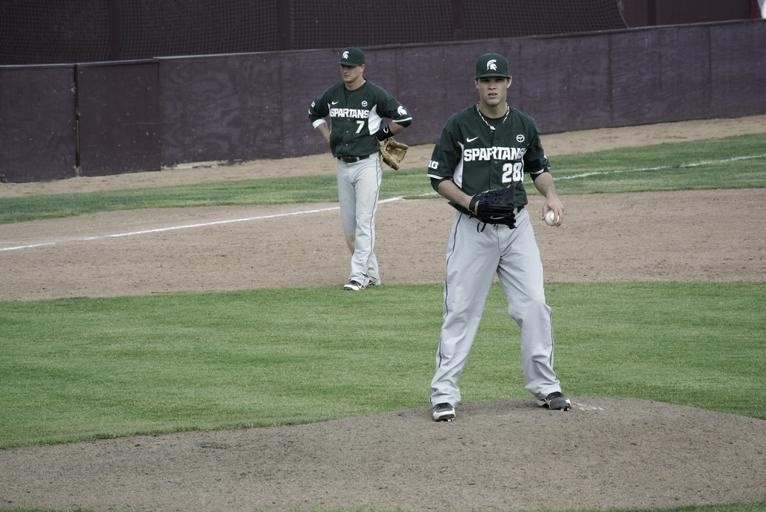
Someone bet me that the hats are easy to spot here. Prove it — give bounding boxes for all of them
[338,48,365,66]
[475,54,510,79]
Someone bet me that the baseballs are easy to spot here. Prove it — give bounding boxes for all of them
[545,210,559,226]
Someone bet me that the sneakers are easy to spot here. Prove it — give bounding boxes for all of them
[344,280,380,291]
[432,402,456,422]
[537,392,570,409]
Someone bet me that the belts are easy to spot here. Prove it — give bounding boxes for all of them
[517,206,524,213]
[337,155,369,163]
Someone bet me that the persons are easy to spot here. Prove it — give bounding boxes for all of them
[427,52,571,422]
[307,47,413,292]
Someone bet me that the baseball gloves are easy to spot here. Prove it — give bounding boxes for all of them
[468,188,516,224]
[377,137,408,170]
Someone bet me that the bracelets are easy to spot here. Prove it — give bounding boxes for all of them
[376,124,394,141]
